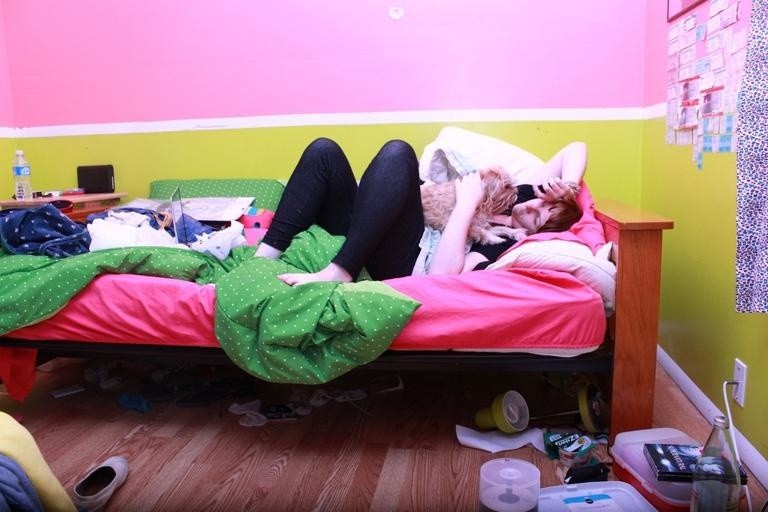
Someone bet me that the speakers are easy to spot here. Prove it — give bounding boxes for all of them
[77,164,115,194]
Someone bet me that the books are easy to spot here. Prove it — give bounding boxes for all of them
[641,443,750,487]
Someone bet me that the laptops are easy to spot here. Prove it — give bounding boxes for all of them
[170,186,188,245]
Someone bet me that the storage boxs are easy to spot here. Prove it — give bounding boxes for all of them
[538,427,747,511]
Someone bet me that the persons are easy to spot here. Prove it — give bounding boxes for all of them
[254,138,588,290]
[0,410,76,511]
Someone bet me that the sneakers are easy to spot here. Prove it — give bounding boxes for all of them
[72,455,128,512]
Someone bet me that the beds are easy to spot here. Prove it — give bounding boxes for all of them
[0,172,678,448]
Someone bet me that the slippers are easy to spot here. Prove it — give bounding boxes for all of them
[176,381,239,408]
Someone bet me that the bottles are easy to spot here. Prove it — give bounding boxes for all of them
[688,415,742,512]
[12,150,34,200]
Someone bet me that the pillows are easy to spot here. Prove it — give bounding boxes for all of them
[484,238,616,318]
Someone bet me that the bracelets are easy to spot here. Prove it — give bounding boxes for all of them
[567,183,582,195]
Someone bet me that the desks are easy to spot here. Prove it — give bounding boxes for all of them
[2,191,130,223]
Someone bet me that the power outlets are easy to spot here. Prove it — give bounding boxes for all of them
[730,357,748,409]
